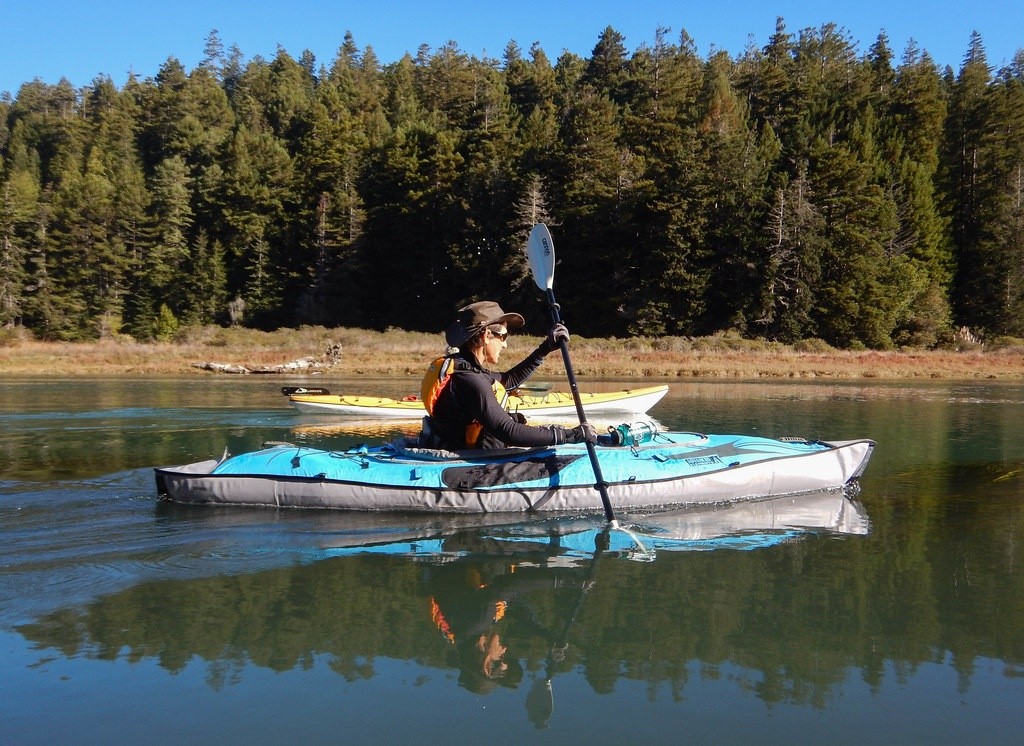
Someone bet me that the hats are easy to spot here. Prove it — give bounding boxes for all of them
[445,300,526,348]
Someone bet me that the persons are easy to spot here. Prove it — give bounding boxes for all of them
[416,300,597,453]
[428,552,599,697]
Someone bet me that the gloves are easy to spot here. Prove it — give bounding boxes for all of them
[539,320,570,358]
[553,421,598,446]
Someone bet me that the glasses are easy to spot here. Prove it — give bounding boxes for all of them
[491,329,510,342]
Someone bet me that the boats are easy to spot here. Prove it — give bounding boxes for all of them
[280,384,672,419]
[155,427,875,513]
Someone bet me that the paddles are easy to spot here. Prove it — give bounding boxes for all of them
[526,222,652,553]
[515,380,553,394]
